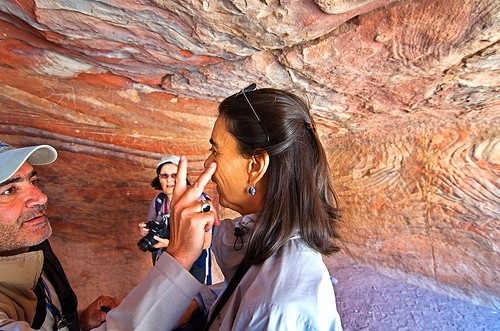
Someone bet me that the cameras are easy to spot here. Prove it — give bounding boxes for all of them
[137,220,170,252]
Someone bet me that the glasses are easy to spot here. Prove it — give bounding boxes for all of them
[158,173,176,179]
[235,83,270,144]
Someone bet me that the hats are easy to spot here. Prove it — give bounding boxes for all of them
[157,155,180,167]
[0,140,58,185]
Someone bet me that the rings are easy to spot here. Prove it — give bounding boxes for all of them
[201,200,210,212]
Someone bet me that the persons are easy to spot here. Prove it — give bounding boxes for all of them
[139,157,212,286]
[0,140,220,331]
[177,87,343,331]
